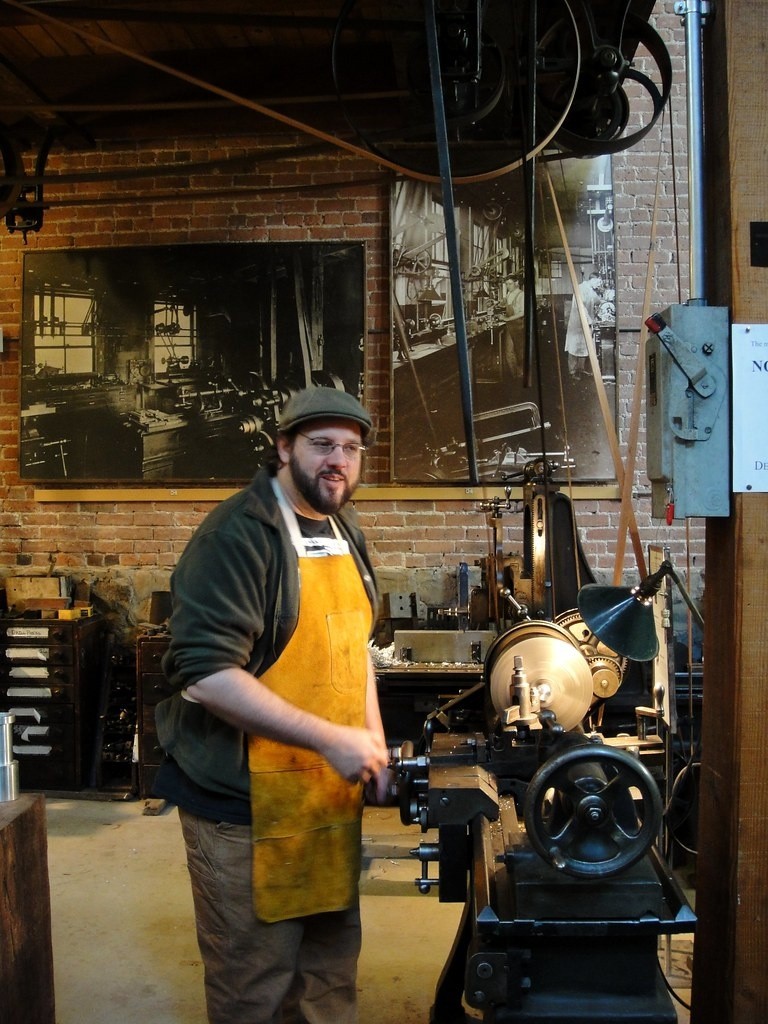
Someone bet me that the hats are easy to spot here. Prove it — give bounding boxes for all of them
[277,387,373,436]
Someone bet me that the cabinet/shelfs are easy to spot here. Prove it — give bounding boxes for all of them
[137,626,173,798]
[0,613,111,791]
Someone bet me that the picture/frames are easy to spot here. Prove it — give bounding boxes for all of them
[18,240,364,484]
[390,150,618,483]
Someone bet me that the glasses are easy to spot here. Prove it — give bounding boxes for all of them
[297,430,370,459]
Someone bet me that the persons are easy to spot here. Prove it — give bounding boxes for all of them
[146,384,392,1024]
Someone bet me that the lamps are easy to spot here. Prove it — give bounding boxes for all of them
[577,560,704,661]
